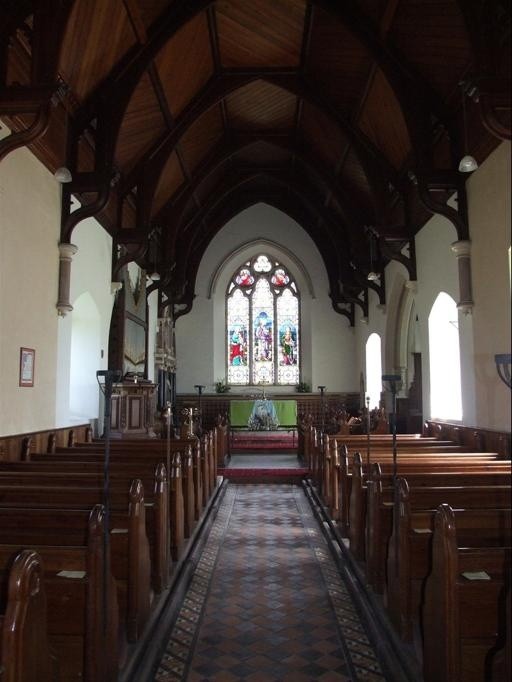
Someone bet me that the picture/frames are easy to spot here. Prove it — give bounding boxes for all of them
[19,345,36,387]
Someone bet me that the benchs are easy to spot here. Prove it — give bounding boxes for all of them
[0,428,218,682]
[304,426,512,682]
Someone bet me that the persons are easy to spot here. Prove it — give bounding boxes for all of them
[280,326,294,367]
[255,317,271,361]
[229,326,245,366]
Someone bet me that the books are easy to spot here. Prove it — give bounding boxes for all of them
[461,568,492,581]
[413,527,433,534]
[110,527,129,534]
[145,501,155,507]
[383,501,395,506]
[57,569,86,579]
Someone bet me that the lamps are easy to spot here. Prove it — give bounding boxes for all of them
[458,85,479,173]
[367,231,378,281]
[55,103,72,183]
[151,236,161,281]
